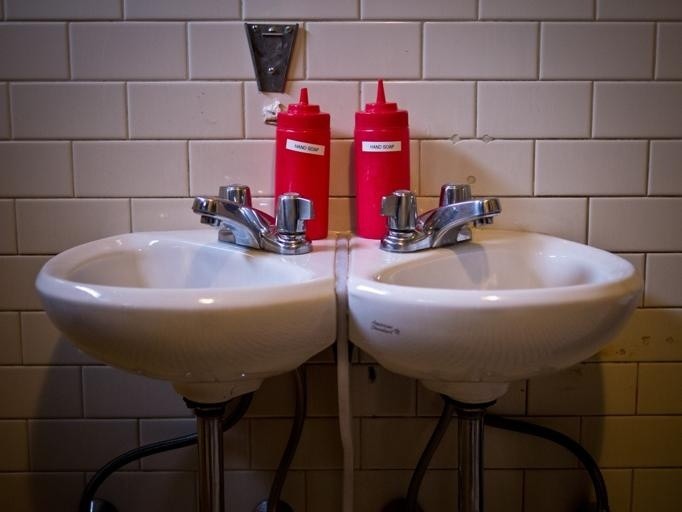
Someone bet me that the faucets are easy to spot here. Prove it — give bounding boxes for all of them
[190,182,314,252]
[377,182,500,252]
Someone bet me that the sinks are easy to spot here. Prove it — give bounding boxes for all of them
[32,226,335,382]
[346,234,632,355]
